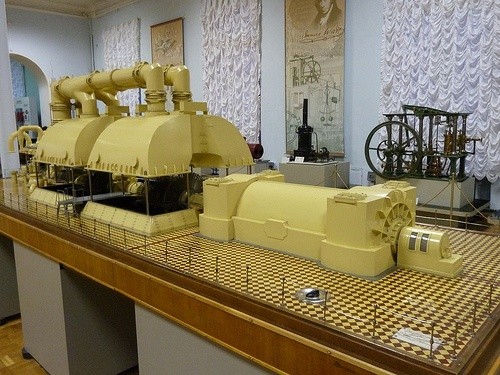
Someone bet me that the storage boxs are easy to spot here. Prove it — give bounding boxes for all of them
[376,173,476,209]
[219,162,268,177]
[279,162,349,189]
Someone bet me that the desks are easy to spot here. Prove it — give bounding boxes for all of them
[414,199,491,227]
[1,177,500,375]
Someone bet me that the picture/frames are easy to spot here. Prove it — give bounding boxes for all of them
[150,17,185,67]
